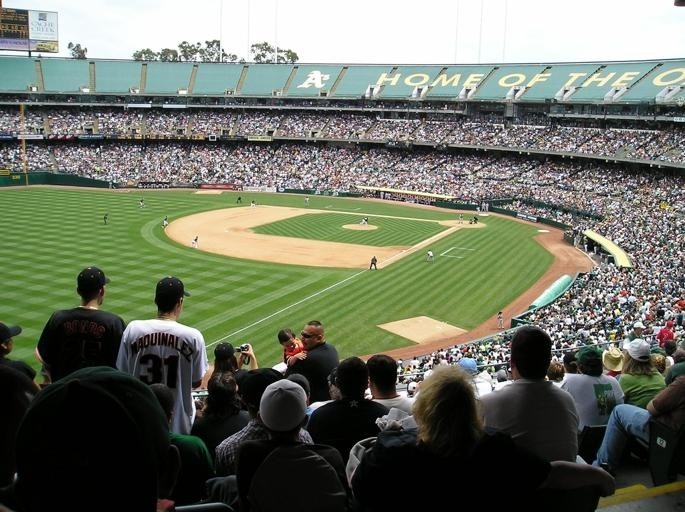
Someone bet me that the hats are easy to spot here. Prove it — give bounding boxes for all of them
[1,267,235,361]
[563,321,674,377]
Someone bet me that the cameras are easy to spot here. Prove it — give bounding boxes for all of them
[236,344,249,352]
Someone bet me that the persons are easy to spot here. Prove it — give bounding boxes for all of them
[0,94,685,512]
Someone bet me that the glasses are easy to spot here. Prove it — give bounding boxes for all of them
[300,330,319,338]
